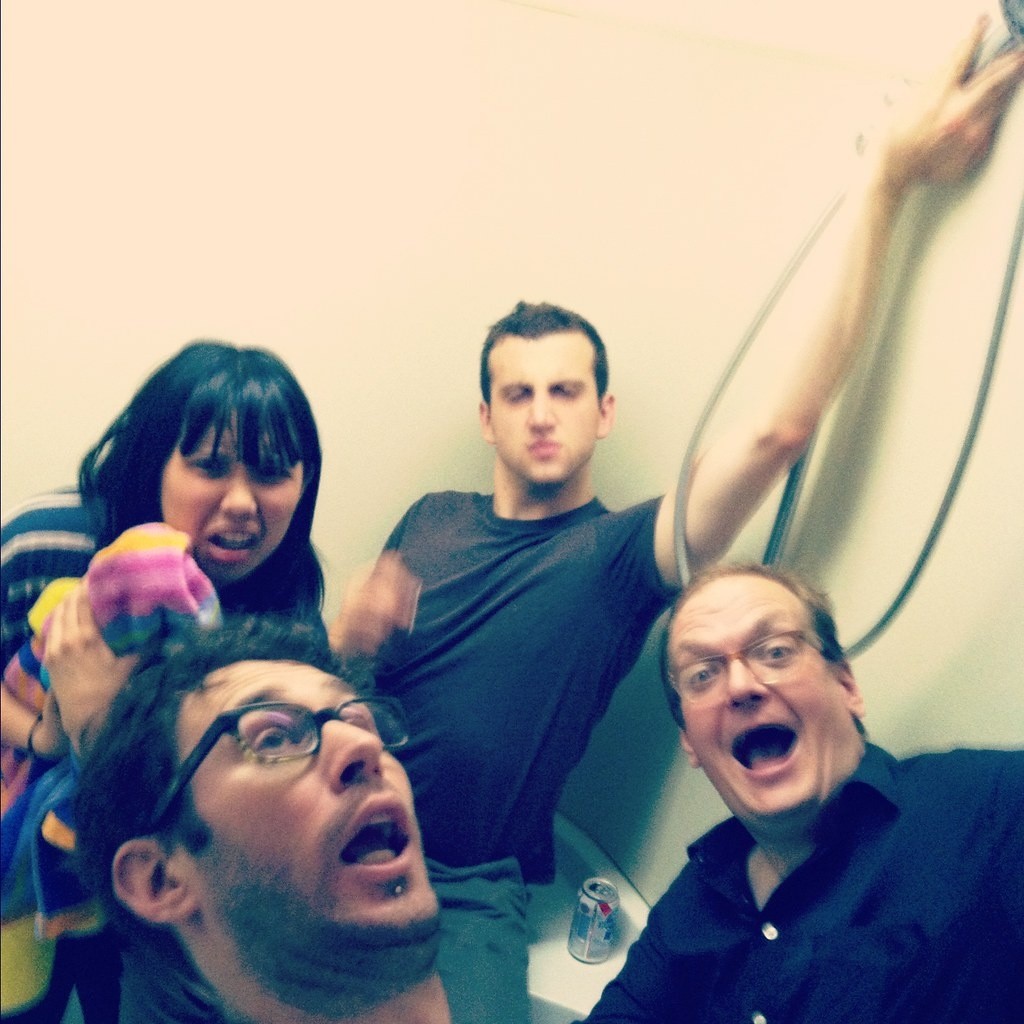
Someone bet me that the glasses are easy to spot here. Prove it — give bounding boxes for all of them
[145,697,413,832]
[671,632,834,700]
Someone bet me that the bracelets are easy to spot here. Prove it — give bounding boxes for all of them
[27,713,44,754]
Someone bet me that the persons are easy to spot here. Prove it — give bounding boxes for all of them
[329,16,1024,1020]
[571,561,1024,1023]
[71,612,457,1023]
[0,337,332,927]
